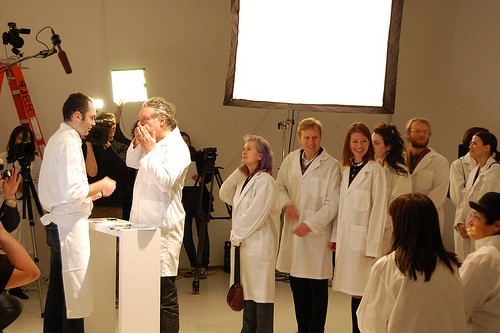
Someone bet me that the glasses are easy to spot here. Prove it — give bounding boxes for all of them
[408,128,430,135]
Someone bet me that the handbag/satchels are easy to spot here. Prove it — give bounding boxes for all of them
[227,282,244,311]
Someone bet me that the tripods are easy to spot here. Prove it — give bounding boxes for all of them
[190,169,232,294]
[18,168,48,317]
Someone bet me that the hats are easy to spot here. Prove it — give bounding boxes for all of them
[469,192,499,222]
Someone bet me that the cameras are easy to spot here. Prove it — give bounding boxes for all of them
[0,159,12,180]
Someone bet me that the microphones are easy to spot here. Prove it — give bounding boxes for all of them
[50,29,72,74]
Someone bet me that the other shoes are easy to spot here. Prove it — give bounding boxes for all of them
[199,268,208,278]
[9,287,28,299]
[184,266,199,277]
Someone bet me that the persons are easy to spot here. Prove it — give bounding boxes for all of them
[455,129,500,264]
[38,94,120,333]
[217,131,282,333]
[271,116,343,333]
[331,121,393,333]
[402,118,450,245]
[368,125,414,208]
[123,97,193,333]
[128,113,213,279]
[450,125,500,264]
[355,193,469,333]
[0,127,47,333]
[451,193,500,333]
[81,108,137,309]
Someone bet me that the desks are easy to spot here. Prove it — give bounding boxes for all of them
[84,217,162,333]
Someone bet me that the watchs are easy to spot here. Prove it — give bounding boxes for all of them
[6,199,16,203]
